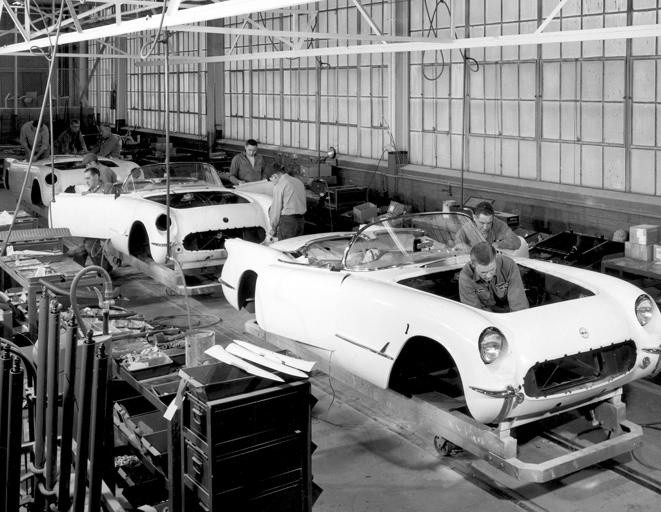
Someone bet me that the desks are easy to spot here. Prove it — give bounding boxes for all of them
[600,257,661,297]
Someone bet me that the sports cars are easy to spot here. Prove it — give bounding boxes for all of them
[2,153,144,219]
[216,212,660,431]
[48,161,271,279]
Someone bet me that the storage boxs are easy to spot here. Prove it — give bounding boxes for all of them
[621,223,661,265]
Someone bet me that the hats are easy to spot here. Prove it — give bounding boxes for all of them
[79,153,96,166]
[264,163,280,182]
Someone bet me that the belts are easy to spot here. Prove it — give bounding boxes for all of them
[281,214,303,218]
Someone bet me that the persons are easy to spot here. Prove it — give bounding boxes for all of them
[228,137,268,187]
[458,241,530,312]
[81,153,120,185]
[456,200,520,254]
[20,119,51,162]
[91,123,120,158]
[56,118,89,156]
[265,161,308,241]
[74,167,117,279]
